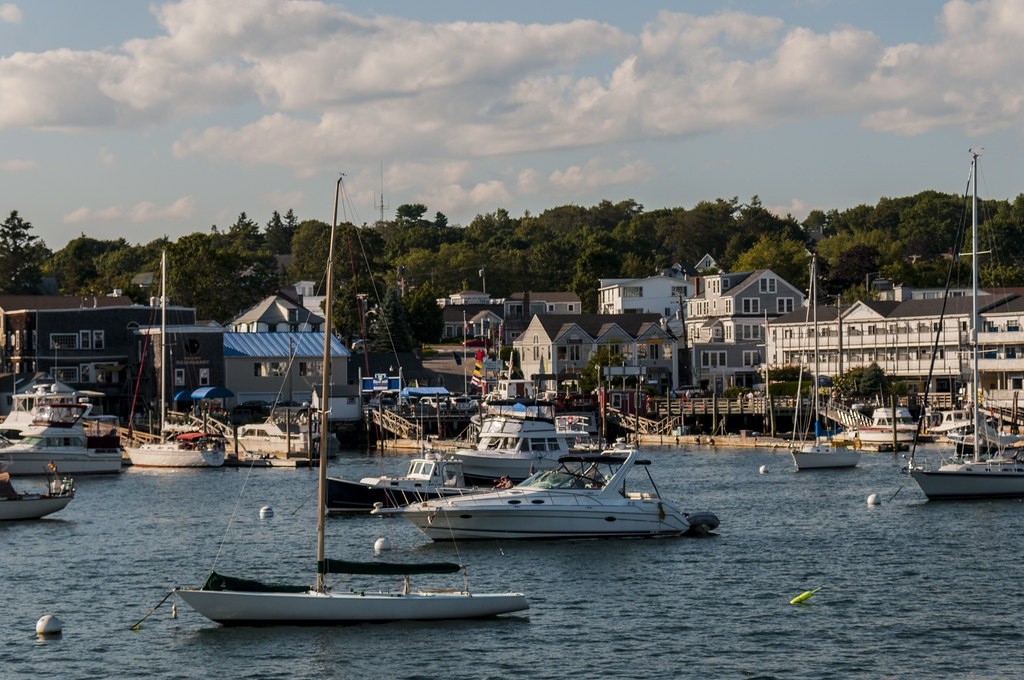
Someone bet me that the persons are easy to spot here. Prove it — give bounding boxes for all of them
[446,397,451,415]
[402,402,410,416]
[671,389,676,398]
[494,475,513,489]
[744,388,764,398]
[686,389,691,398]
[614,400,620,407]
[646,395,650,410]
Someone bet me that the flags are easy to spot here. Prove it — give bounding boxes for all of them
[475,351,484,363]
[464,317,469,335]
[499,325,503,337]
[471,375,482,385]
[453,351,461,365]
[473,362,482,375]
[530,463,534,476]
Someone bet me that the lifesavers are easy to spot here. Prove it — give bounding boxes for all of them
[39,408,44,413]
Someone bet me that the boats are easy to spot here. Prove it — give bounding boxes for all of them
[1,460,78,523]
[0,342,722,541]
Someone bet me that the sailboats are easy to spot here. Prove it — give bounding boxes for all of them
[784,250,866,470]
[833,150,1024,500]
[165,172,534,623]
